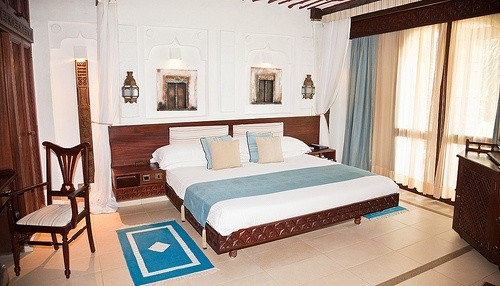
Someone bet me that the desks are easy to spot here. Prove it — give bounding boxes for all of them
[0,169,23,276]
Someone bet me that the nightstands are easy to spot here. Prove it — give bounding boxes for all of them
[311,147,336,160]
[112,164,165,201]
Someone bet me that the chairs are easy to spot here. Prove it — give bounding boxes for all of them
[15,141,95,280]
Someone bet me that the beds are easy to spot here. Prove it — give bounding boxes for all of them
[108,116,399,257]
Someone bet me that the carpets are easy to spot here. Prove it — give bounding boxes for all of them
[364,205,407,222]
[115,220,220,286]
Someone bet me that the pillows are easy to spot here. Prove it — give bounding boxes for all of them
[152,140,207,171]
[238,136,312,164]
[256,136,283,163]
[209,139,240,169]
[246,132,273,162]
[201,134,232,169]
[233,122,284,137]
[168,124,229,144]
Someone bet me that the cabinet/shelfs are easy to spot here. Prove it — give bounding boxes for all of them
[0,31,46,257]
[452,147,500,270]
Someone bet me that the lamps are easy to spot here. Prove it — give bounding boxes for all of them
[170,47,182,61]
[262,50,274,64]
[301,74,316,99]
[122,70,140,104]
